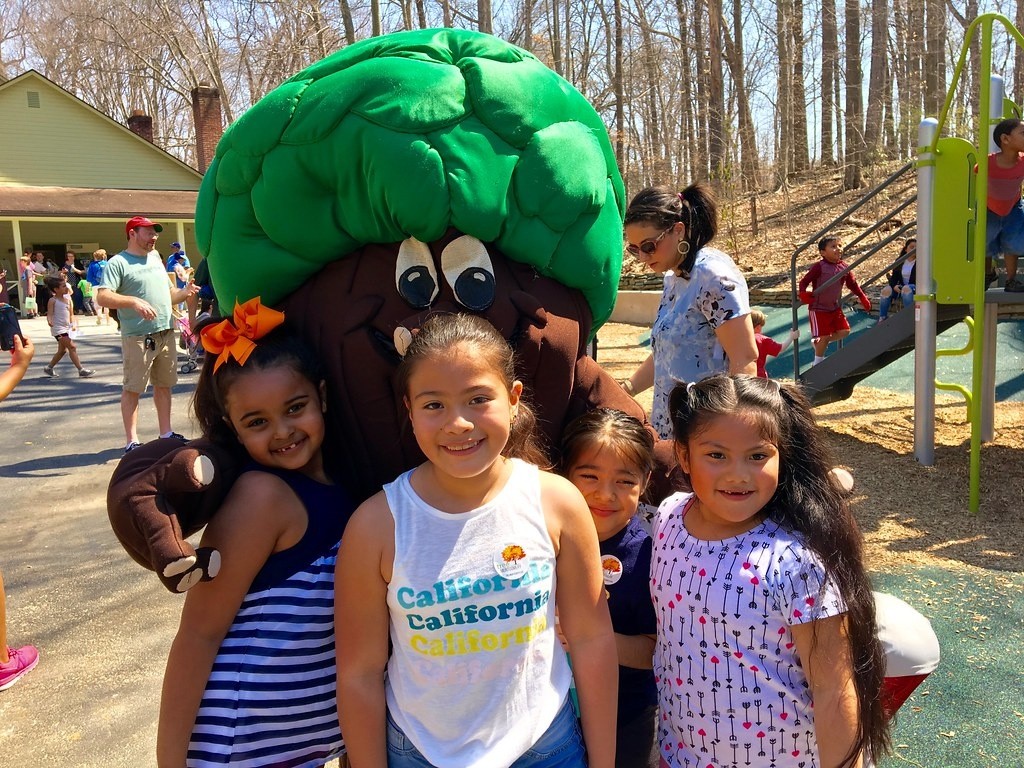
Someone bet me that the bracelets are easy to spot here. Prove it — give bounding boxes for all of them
[624,380,635,397]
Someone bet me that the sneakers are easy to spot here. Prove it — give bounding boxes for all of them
[1004,279,1024,292]
[44,367,59,377]
[0,645,39,694]
[79,368,95,378]
[985,267,998,290]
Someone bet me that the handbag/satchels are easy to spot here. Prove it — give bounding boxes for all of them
[25,296,35,309]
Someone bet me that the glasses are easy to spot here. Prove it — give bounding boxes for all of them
[136,228,159,238]
[626,223,675,256]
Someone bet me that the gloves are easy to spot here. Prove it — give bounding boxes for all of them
[799,292,816,304]
[859,294,871,312]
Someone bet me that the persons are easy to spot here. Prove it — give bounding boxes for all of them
[879,239,916,321]
[553,408,657,768]
[97,217,201,455]
[870,587,941,721]
[973,118,1024,292]
[800,233,872,367]
[155,296,346,767]
[750,310,800,379]
[0,302,39,691]
[43,277,95,378]
[334,314,619,767]
[0,242,110,325]
[623,182,759,440]
[160,242,213,355]
[649,372,893,768]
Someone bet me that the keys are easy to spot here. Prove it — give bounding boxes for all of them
[146,338,155,351]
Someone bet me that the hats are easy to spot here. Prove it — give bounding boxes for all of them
[174,252,186,259]
[170,242,180,249]
[125,217,163,234]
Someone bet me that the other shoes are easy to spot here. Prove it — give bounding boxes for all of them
[96,317,102,325]
[85,312,94,316]
[123,440,143,453]
[27,313,34,319]
[158,432,192,444]
[34,313,39,318]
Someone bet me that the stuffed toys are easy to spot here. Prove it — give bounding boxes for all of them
[106,26,687,594]
